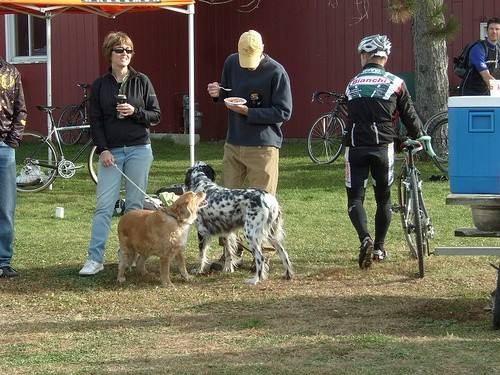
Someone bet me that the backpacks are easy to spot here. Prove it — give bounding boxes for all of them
[454,40,488,78]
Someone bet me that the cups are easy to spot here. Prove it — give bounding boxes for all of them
[56,206,64,219]
[489,80,500,97]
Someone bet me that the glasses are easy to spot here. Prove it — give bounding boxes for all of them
[112,47,132,54]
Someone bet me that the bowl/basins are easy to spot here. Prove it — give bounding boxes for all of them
[224,97,248,106]
[471,204,500,231]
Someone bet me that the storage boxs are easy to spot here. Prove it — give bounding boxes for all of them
[448,96,500,193]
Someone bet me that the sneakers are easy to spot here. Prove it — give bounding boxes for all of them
[372,248,387,261]
[250,257,270,272]
[0,266,18,279]
[211,254,243,273]
[79,260,104,275]
[118,258,136,270]
[359,236,373,269]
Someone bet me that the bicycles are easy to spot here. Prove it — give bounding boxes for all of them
[57,83,90,145]
[394,134,448,277]
[307,91,348,164]
[16,105,99,192]
[422,107,448,174]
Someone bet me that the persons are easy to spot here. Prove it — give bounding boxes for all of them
[343,34,427,271]
[206,30,293,274]
[79,31,162,275]
[0,56,27,276]
[459,18,500,98]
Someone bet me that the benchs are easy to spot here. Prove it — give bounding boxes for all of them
[454,227,499,237]
[434,247,500,256]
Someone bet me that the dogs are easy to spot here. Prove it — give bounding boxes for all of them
[117,191,209,287]
[185,162,296,285]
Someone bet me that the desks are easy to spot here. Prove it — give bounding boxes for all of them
[446,195,500,330]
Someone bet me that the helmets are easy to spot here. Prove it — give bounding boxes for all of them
[357,34,392,55]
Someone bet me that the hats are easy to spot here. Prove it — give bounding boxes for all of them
[238,30,263,69]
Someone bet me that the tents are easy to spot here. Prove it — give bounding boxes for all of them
[0,0,196,168]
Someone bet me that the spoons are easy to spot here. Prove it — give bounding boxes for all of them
[217,86,231,91]
